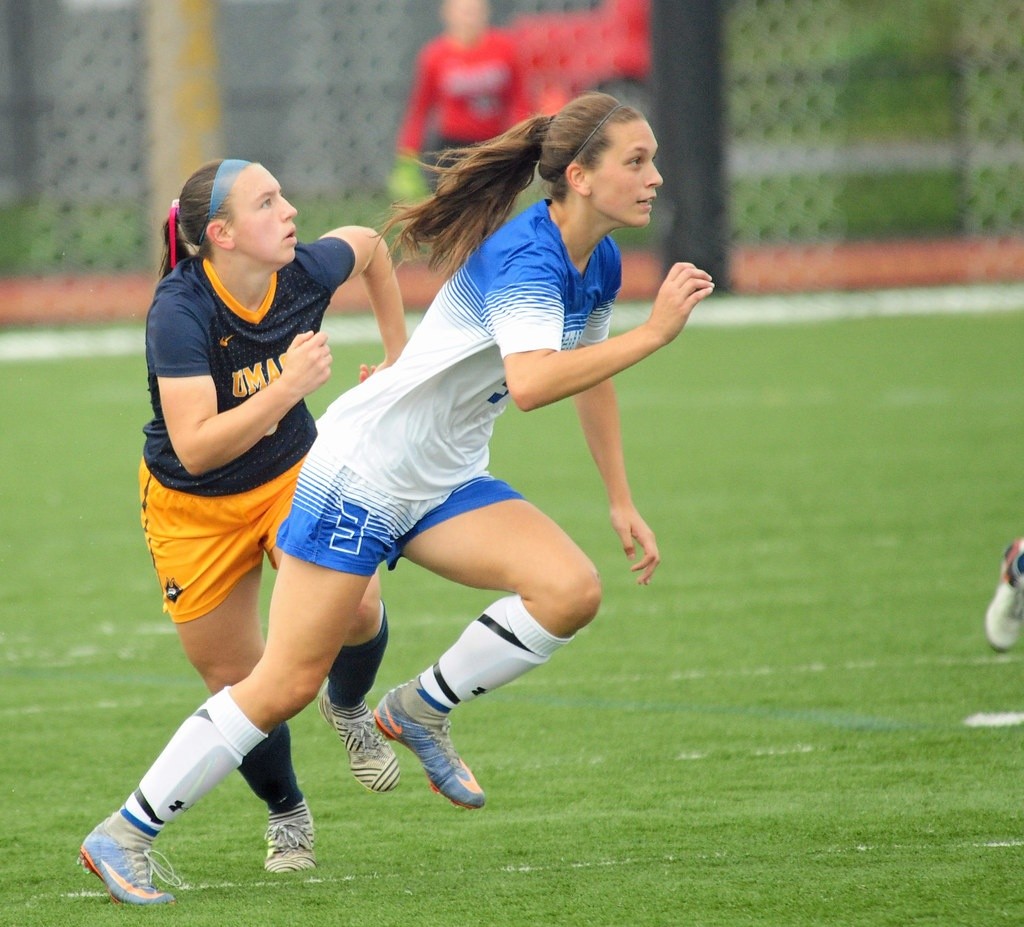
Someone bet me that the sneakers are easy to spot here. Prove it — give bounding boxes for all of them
[985,541,1024,652]
[77,819,182,905]
[372,681,485,809]
[318,681,401,791]
[264,800,316,874]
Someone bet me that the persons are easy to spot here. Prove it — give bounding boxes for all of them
[388,0,535,205]
[985,539,1024,652]
[78,92,715,904]
[138,159,407,876]
